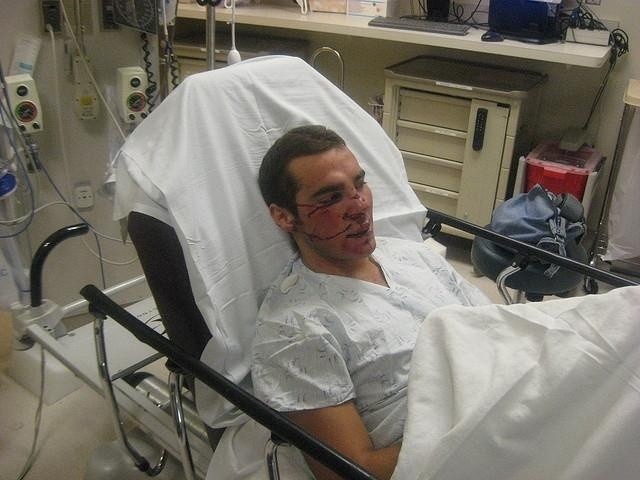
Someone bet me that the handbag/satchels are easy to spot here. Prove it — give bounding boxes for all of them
[495,182,589,279]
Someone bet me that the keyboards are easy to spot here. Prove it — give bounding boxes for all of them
[368,16,470,35]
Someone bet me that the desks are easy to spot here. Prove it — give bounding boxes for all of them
[175,5,612,69]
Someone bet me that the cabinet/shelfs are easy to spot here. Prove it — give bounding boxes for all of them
[381,56,547,241]
[160,31,309,96]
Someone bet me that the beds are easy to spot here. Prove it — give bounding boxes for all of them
[30,54,640,479]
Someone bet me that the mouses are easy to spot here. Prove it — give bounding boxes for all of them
[480,31,505,41]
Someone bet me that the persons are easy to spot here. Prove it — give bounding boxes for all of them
[251,125,495,480]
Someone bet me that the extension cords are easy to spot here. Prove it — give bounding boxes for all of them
[565,27,610,47]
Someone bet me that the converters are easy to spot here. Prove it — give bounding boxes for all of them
[558,128,589,151]
